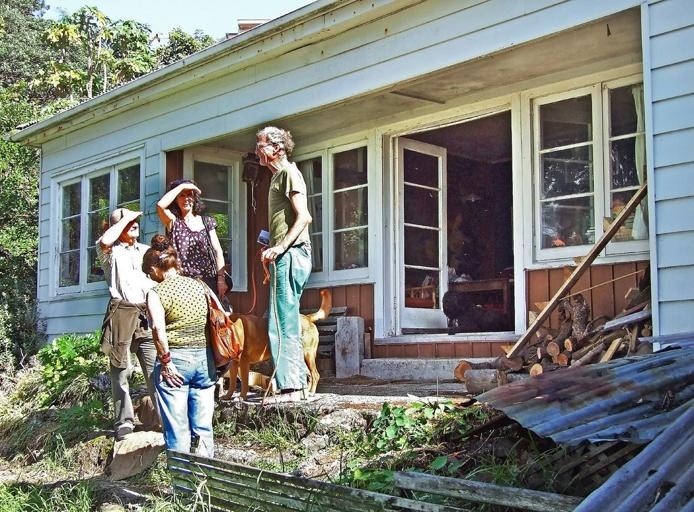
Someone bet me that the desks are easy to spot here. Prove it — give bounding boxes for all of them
[442,274,514,315]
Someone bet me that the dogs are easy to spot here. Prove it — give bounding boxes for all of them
[219,289,333,402]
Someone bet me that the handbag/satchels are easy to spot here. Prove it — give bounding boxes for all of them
[209,310,241,367]
[215,270,232,292]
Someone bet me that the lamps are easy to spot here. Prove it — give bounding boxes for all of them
[485,222,514,245]
[467,189,482,204]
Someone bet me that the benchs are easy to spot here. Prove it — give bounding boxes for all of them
[262,306,349,370]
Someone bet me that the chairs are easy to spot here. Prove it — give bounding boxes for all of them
[404,282,438,311]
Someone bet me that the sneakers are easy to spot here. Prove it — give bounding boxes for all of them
[116,427,132,441]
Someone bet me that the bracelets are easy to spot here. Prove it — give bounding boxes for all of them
[159,351,171,364]
[217,282,224,283]
[218,275,224,277]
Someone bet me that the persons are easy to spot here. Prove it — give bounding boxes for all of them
[157,179,228,302]
[254,126,313,405]
[142,233,220,460]
[95,207,160,436]
